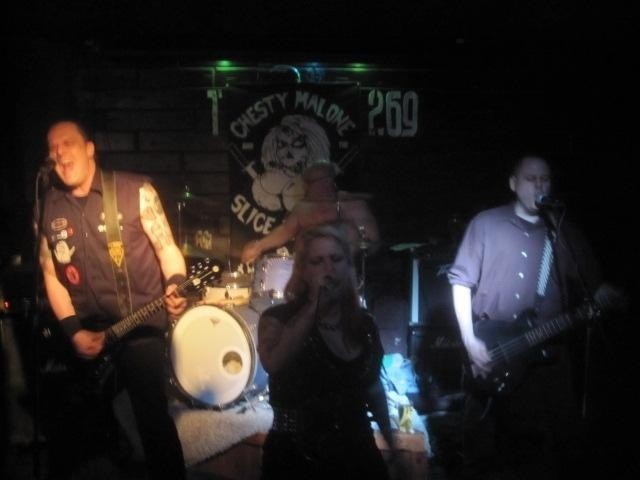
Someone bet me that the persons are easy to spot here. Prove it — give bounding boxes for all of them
[39,121,189,480]
[446,154,614,378]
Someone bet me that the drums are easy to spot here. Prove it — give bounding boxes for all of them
[164,301,267,410]
[248,253,294,313]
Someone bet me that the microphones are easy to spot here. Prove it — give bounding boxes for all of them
[535,192,567,211]
[318,272,333,293]
[36,157,57,177]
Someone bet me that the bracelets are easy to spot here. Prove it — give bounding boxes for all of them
[60,315,83,339]
[167,273,185,287]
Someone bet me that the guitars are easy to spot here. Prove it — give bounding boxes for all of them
[29,257,221,406]
[469,295,613,385]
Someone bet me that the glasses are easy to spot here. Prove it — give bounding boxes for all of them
[304,175,331,185]
